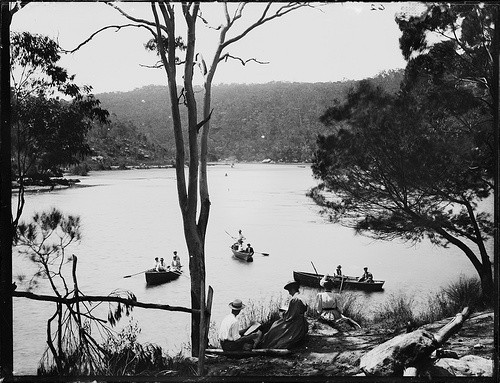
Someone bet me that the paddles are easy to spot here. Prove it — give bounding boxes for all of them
[255,252,269,257]
[123,271,146,278]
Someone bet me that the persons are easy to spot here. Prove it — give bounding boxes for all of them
[232,229,246,245]
[258,280,308,349]
[219,299,258,362]
[334,265,342,280]
[315,273,357,321]
[236,240,244,251]
[358,266,373,283]
[246,243,253,252]
[153,251,183,272]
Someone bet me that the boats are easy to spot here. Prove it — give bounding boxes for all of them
[293,269,385,291]
[145,266,183,283]
[231,242,254,260]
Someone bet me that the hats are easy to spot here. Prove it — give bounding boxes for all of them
[284,281,300,289]
[229,299,245,310]
